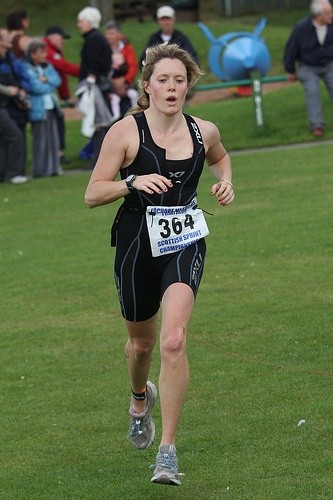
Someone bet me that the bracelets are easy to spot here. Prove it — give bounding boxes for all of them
[218,179,235,189]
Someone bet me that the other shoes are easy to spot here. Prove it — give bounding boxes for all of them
[313,128,323,136]
[9,175,28,184]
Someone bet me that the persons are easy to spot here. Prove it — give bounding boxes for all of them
[282,0,333,136]
[85,45,234,485]
[0,6,198,186]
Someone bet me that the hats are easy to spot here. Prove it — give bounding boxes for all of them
[157,6,175,18]
[46,26,69,38]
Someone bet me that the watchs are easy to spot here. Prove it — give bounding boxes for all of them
[124,174,138,192]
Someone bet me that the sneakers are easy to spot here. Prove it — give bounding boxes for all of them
[151,445,181,486]
[129,380,157,449]
